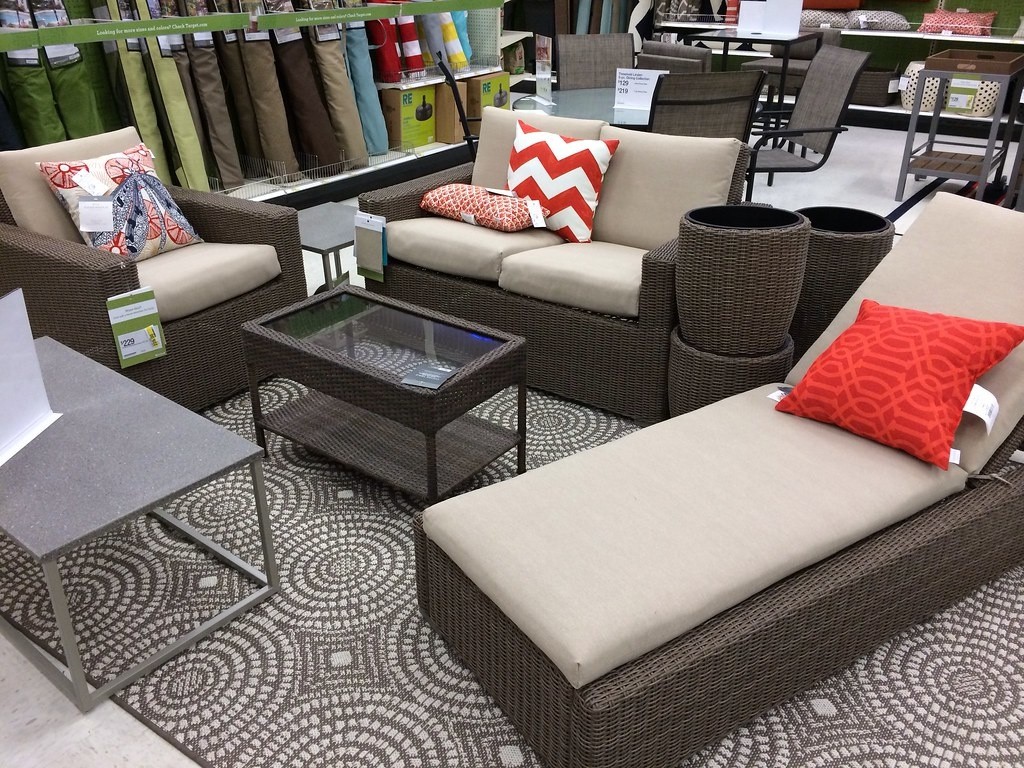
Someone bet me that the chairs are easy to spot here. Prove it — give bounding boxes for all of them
[403,191,1024,768]
[556,33,636,90]
[635,29,870,200]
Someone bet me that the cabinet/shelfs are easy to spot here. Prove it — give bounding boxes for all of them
[895,70,1024,213]
[224,0,558,209]
[659,0,1024,142]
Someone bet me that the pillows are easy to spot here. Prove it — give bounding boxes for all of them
[773,300,1023,469]
[419,185,550,232]
[501,121,622,243]
[36,144,202,266]
[1013,15,1024,40]
[918,9,998,36]
[801,10,911,32]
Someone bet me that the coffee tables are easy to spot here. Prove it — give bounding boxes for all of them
[237,283,531,508]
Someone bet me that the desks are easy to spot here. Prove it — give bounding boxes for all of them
[684,30,825,187]
[513,89,615,123]
[0,338,286,712]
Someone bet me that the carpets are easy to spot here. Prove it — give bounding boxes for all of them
[0,339,1024,768]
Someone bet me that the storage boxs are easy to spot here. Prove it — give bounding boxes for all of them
[377,72,512,150]
[926,50,1024,75]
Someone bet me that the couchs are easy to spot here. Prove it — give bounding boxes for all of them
[360,105,754,430]
[0,126,308,414]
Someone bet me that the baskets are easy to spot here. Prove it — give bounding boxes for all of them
[849,62,900,107]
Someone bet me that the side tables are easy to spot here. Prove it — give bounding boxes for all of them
[297,202,359,289]
